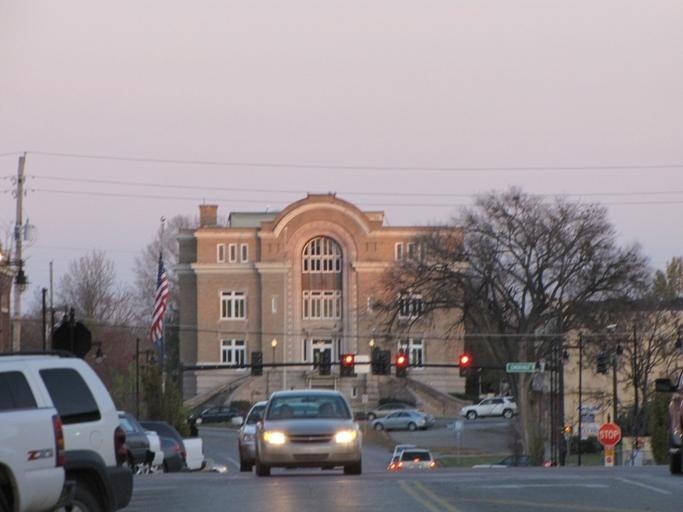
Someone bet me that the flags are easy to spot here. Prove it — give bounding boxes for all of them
[148,254,168,344]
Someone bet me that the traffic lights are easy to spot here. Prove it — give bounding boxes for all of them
[340,354,353,376]
[396,355,406,376]
[460,355,468,376]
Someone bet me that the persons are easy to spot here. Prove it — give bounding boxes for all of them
[189,418,201,435]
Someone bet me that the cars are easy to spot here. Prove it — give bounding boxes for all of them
[253,388,367,476]
[367,403,424,419]
[387,444,435,471]
[116,409,206,471]
[472,453,530,467]
[185,406,244,425]
[474,396,514,404]
[368,411,435,431]
[231,401,322,473]
[654,368,682,475]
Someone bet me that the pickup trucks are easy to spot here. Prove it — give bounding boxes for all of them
[0,408,76,512]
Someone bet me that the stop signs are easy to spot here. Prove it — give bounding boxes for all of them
[598,423,620,446]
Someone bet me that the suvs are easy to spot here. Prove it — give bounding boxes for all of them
[459,397,519,420]
[0,352,133,512]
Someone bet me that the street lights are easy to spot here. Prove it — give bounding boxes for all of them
[134,336,157,420]
[41,286,69,352]
[561,333,583,469]
[607,323,639,450]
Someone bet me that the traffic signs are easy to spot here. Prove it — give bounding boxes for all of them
[506,362,544,372]
